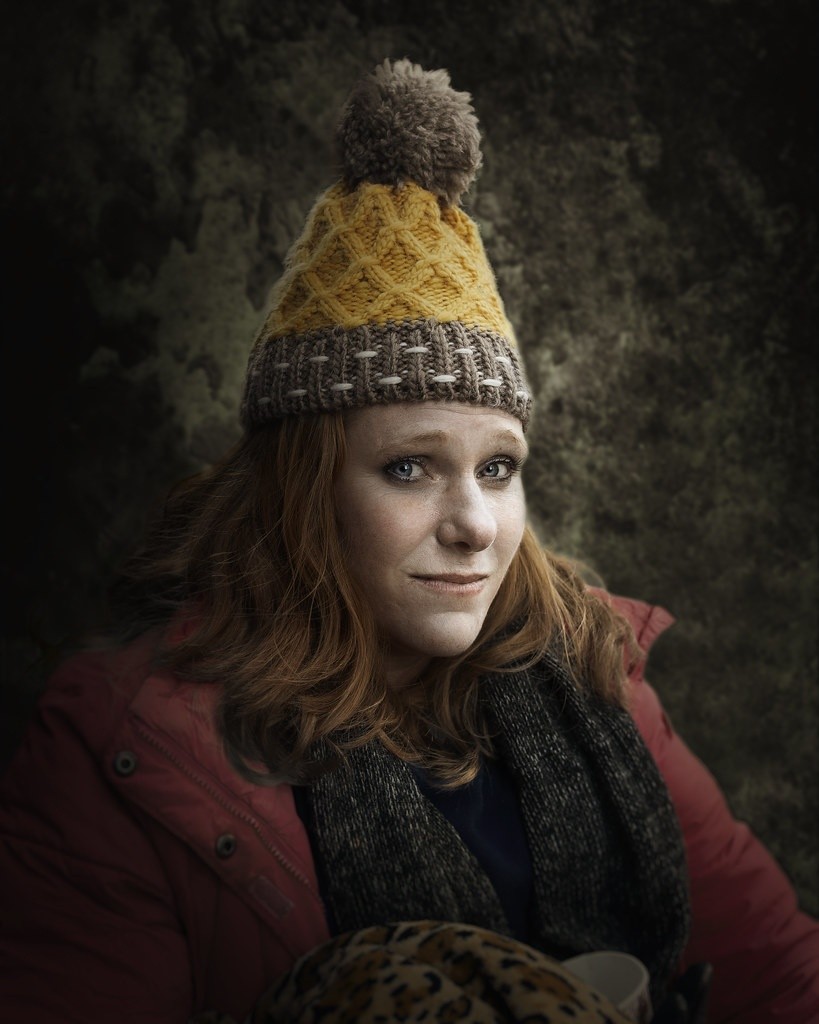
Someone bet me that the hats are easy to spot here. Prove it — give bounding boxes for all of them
[241,57,533,421]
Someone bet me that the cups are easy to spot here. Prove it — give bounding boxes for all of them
[561,951,649,1023]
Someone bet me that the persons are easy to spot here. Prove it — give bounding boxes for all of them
[0,57,819,1024]
